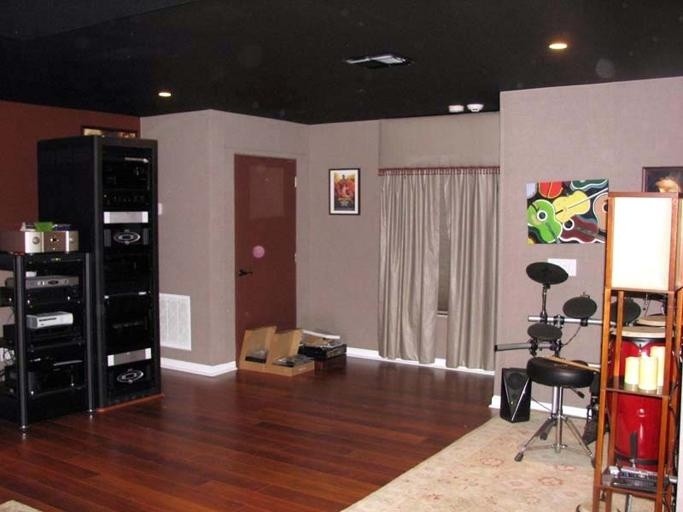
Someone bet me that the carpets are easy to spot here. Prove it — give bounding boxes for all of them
[339,410,676,512]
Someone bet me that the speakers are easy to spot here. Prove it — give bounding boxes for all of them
[0,229,45,255]
[501,367,532,422]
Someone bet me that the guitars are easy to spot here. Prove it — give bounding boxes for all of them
[526,180,607,245]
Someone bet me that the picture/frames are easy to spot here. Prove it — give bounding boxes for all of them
[642,166,683,200]
[80,125,137,138]
[328,168,361,216]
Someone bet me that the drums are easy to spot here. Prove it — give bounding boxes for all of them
[610,300,640,324]
[526,262,568,284]
[607,326,674,471]
[564,297,597,318]
[528,324,561,340]
[634,314,667,327]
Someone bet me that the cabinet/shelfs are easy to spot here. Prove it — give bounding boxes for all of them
[37,137,161,430]
[0,250,96,433]
[592,190,683,512]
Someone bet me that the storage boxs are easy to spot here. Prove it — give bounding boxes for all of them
[239,325,347,376]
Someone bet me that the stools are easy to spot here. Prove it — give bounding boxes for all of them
[513,357,596,468]
